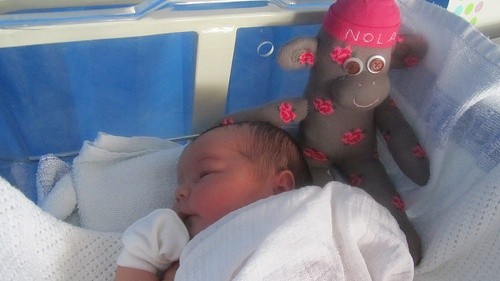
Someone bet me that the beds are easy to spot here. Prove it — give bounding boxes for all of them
[0,1,500,281]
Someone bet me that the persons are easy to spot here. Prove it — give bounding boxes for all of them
[116,121,414,281]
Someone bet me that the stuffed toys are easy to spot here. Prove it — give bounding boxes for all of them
[214,0,429,265]
